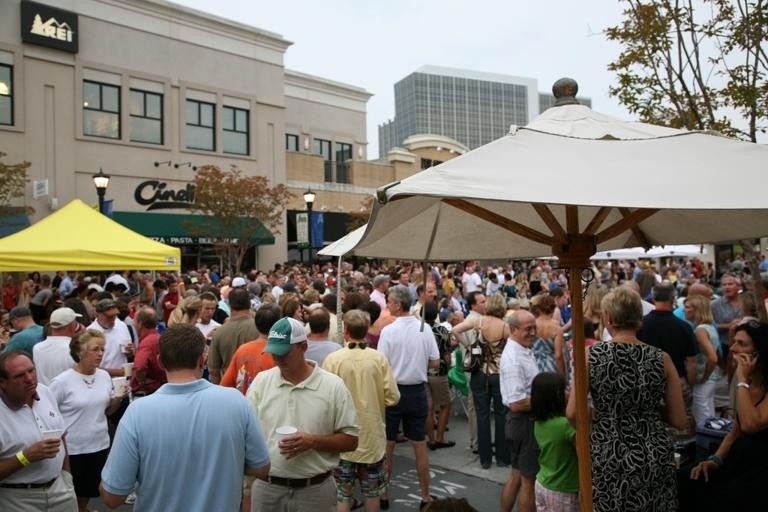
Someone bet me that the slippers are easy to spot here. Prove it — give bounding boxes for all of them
[352,497,363,508]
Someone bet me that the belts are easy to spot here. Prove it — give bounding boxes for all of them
[1,477,59,490]
[262,470,336,489]
[427,372,441,376]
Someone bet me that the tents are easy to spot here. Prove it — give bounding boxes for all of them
[0,196,182,282]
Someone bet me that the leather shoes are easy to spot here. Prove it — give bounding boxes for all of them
[427,441,435,448]
[438,440,456,448]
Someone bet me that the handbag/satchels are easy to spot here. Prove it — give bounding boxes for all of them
[461,314,483,371]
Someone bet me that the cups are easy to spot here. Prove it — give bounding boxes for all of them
[123,363,134,376]
[42,429,63,451]
[111,377,126,397]
[120,344,128,354]
[276,426,297,458]
[75,321,82,333]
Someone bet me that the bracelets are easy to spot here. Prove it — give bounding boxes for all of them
[708,455,722,466]
[736,382,750,389]
[15,450,30,466]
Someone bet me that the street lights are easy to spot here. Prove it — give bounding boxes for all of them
[302,186,317,268]
[92,166,110,287]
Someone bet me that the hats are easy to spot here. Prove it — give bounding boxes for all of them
[184,296,201,311]
[6,307,31,324]
[51,307,83,327]
[96,298,120,317]
[261,316,306,357]
[232,277,245,286]
[373,274,388,287]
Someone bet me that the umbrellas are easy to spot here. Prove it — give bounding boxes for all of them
[315,77,768,511]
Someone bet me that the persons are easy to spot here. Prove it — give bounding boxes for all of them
[2,306,44,354]
[458,291,486,453]
[219,302,283,396]
[731,292,758,332]
[566,286,687,512]
[208,289,258,384]
[529,295,565,373]
[499,310,539,512]
[0,258,569,304]
[167,296,202,327]
[306,295,344,343]
[580,255,768,317]
[86,299,135,379]
[530,372,579,512]
[322,309,400,512]
[637,284,701,404]
[244,317,359,512]
[47,329,129,512]
[303,308,342,369]
[683,294,722,427]
[377,284,440,509]
[196,292,222,381]
[419,302,456,450]
[712,276,742,364]
[452,294,509,469]
[0,350,79,512]
[128,306,166,398]
[689,320,768,512]
[33,307,83,386]
[99,323,271,512]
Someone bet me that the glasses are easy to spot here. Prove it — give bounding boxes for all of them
[348,341,368,349]
[737,318,761,329]
[89,345,105,353]
